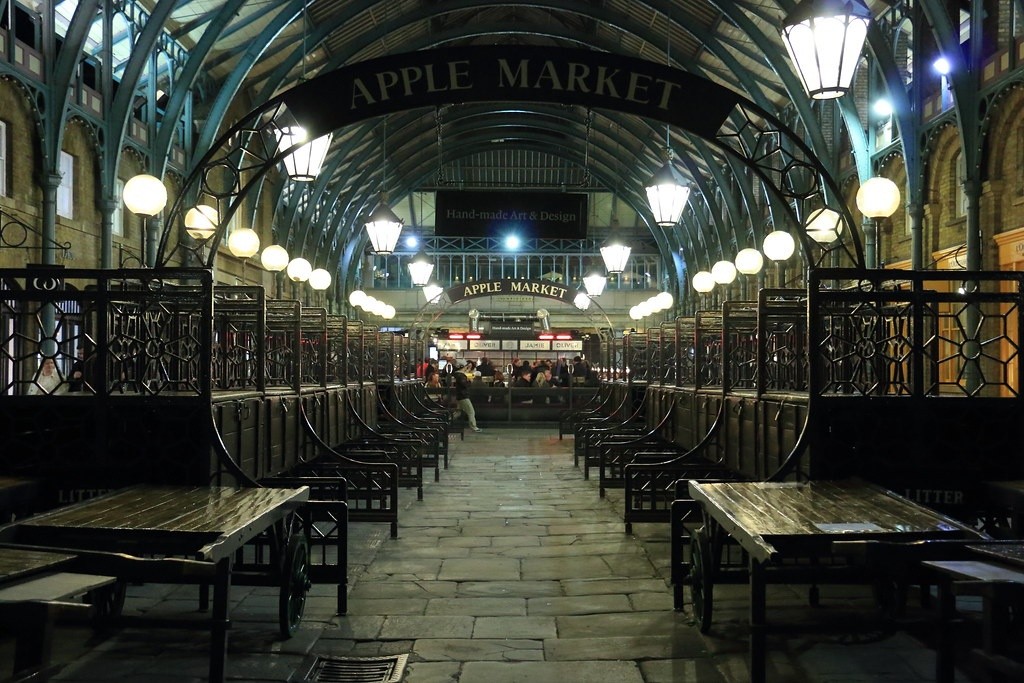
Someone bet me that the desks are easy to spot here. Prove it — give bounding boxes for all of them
[441,376,494,387]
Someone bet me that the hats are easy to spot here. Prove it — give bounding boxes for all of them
[455,360,467,368]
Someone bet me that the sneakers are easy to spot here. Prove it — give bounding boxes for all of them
[473,427,482,432]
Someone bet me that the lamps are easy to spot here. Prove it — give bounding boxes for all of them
[123,174,168,269]
[735,248,763,300]
[261,244,289,299]
[287,258,312,283]
[856,176,901,269]
[269,0,334,182]
[309,269,331,291]
[349,290,367,319]
[643,0,692,227]
[361,296,377,312]
[712,260,736,301]
[184,205,222,266]
[404,330,409,338]
[574,291,591,312]
[763,231,796,288]
[647,296,661,327]
[805,208,843,268]
[228,228,260,286]
[422,276,444,305]
[600,112,632,274]
[692,271,715,311]
[382,305,396,331]
[656,292,673,321]
[629,305,644,333]
[407,190,435,287]
[582,111,606,297]
[638,301,652,333]
[362,115,405,254]
[372,301,386,316]
[775,0,874,100]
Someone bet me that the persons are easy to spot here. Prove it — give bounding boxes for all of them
[449,358,484,433]
[395,354,620,408]
